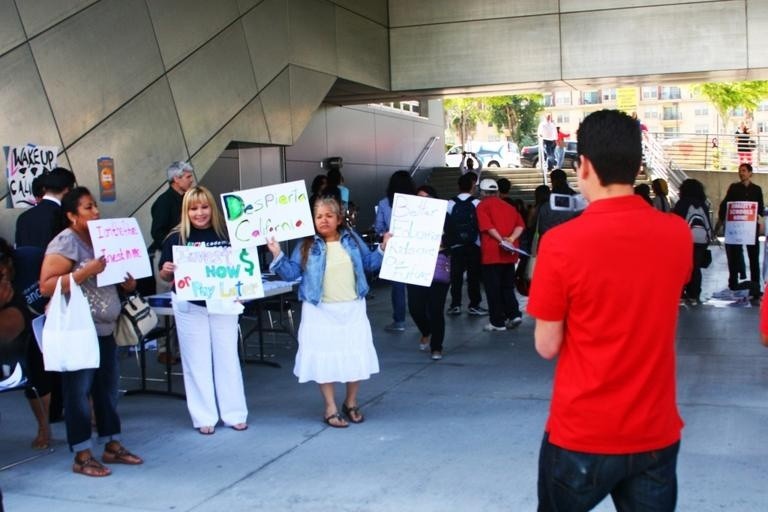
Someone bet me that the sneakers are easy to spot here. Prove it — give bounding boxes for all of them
[687,298,697,306]
[446,305,460,314]
[506,317,522,329]
[483,323,506,331]
[383,320,405,331]
[713,289,733,298]
[468,306,489,315]
[727,299,752,308]
[680,299,688,311]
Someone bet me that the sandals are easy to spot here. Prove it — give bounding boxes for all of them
[31,426,51,449]
[73,455,112,477]
[102,444,144,464]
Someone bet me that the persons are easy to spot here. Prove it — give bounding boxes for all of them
[264,194,394,428]
[537,112,558,172]
[734,121,753,165]
[555,125,571,169]
[0,237,52,450]
[525,109,695,512]
[0,252,30,357]
[450,195,479,244]
[672,179,714,309]
[497,178,518,210]
[158,184,249,435]
[446,172,490,317]
[714,162,765,299]
[407,185,453,360]
[309,174,329,218]
[151,161,196,366]
[323,168,351,216]
[374,170,419,332]
[475,178,526,331]
[537,169,578,235]
[710,137,721,170]
[39,185,144,477]
[14,166,77,424]
[759,282,768,346]
[459,151,483,185]
[527,184,550,229]
[651,177,672,212]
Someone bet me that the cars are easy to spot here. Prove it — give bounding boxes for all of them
[519,140,578,170]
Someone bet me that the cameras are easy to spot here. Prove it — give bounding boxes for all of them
[549,191,590,213]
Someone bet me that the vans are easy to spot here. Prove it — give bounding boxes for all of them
[445,141,521,170]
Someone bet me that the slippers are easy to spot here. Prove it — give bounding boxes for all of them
[342,403,364,423]
[323,411,349,427]
[199,425,215,434]
[231,423,248,430]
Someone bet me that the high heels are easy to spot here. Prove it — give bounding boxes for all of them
[432,355,442,360]
[419,334,430,350]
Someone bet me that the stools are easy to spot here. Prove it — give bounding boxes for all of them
[0,383,55,472]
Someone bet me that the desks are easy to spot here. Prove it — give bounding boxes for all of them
[124,274,292,402]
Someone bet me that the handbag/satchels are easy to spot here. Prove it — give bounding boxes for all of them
[113,284,158,347]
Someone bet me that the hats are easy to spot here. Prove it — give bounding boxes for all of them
[479,178,498,191]
